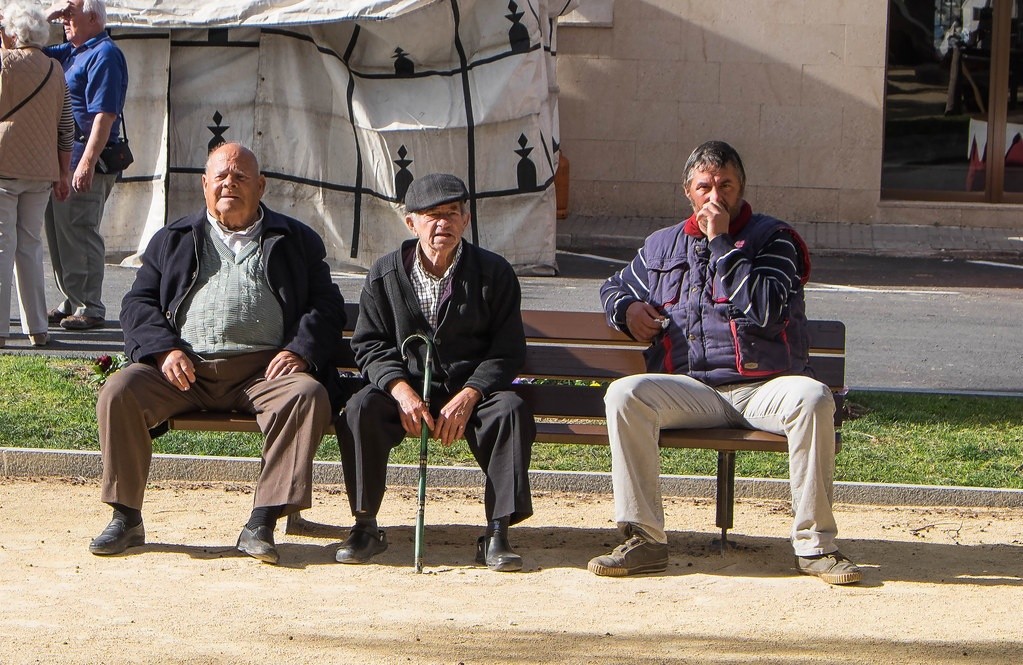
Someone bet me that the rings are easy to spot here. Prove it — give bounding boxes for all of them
[459,426,464,428]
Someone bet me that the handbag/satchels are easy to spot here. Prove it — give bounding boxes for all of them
[94,138,134,174]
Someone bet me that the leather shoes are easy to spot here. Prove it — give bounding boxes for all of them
[237,525,278,564]
[89,518,146,554]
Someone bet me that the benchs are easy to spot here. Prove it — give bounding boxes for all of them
[149,304,846,548]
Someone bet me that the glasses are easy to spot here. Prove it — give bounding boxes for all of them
[59,12,84,20]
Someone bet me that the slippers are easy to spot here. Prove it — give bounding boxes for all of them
[477,536,523,570]
[334,524,388,563]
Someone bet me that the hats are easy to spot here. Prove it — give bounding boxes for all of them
[406,173,467,211]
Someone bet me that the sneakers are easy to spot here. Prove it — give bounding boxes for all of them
[794,549,862,583]
[587,534,668,576]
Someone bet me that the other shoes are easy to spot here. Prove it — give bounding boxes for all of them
[28,333,51,346]
[60,315,104,329]
[47,308,70,323]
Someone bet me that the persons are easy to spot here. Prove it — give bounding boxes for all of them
[336,173,535,570]
[0,0,127,347]
[586,141,862,584]
[89,143,347,564]
